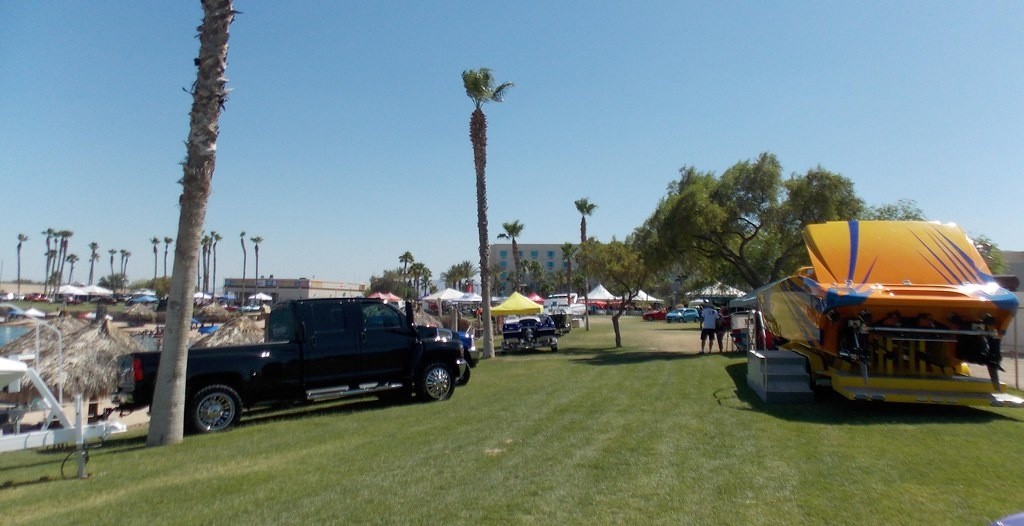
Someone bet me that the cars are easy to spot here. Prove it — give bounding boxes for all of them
[642,309,669,320]
[666,307,700,324]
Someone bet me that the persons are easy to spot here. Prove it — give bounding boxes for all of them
[698,302,730,355]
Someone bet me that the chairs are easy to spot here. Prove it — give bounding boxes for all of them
[731,332,755,353]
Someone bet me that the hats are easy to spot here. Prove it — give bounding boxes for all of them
[721,307,728,311]
[705,301,716,307]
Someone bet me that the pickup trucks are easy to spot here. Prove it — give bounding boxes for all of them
[109,295,471,433]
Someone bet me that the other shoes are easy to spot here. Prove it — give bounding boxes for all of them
[699,352,705,355]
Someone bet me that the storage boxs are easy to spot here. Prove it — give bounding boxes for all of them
[730,313,755,329]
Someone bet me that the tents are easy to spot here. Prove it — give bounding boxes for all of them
[54,284,272,305]
[578,284,664,317]
[367,286,546,334]
